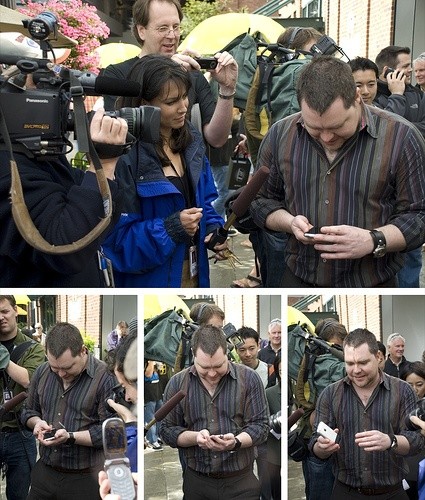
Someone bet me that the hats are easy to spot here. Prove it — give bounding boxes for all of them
[0,30,71,80]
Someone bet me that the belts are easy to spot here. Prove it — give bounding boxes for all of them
[201,466,252,478]
[347,483,404,495]
[44,462,100,475]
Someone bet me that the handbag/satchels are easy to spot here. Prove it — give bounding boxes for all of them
[289,328,310,382]
[225,185,259,234]
[210,33,311,123]
[228,141,250,190]
[287,425,311,462]
[144,309,196,370]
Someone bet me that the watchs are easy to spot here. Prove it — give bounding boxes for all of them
[370,230,386,259]
[63,433,75,445]
[230,438,241,451]
[387,433,398,452]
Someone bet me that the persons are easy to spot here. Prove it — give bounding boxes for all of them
[107,330,137,472]
[190,302,225,329]
[0,295,44,500]
[100,1,238,148]
[98,55,223,287]
[160,325,270,500]
[400,361,425,399]
[409,416,425,435]
[98,470,137,500]
[107,321,129,352]
[202,108,237,237]
[1,31,128,288]
[308,329,425,500]
[145,360,163,449]
[412,52,425,94]
[32,323,46,345]
[20,322,118,500]
[258,318,281,363]
[296,318,347,500]
[275,26,324,63]
[378,341,386,370]
[265,355,281,499]
[250,55,425,286]
[234,327,269,389]
[346,55,379,108]
[385,333,411,378]
[372,46,425,140]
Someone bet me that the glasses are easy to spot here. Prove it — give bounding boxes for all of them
[150,25,188,38]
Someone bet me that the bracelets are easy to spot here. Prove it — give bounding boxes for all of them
[219,91,236,99]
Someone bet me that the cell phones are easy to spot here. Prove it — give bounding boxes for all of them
[101,418,136,500]
[208,433,222,441]
[314,420,338,443]
[191,59,217,70]
[304,225,326,237]
[43,427,57,438]
[383,68,404,79]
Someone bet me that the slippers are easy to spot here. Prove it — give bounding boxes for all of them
[230,275,263,288]
